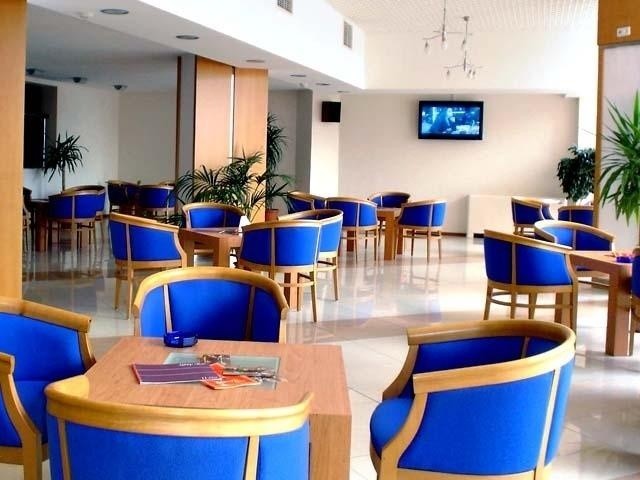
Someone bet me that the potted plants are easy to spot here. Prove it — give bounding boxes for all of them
[266,111,303,220]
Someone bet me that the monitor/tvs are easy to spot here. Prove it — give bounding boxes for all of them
[418,100,484,140]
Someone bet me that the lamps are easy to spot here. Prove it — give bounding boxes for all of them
[443,15,489,82]
[421,0,450,54]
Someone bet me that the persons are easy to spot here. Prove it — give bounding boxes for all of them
[430,106,456,134]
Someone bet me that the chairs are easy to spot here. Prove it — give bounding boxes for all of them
[364,191,412,249]
[558,205,596,229]
[236,219,322,322]
[534,219,618,276]
[1,295,96,480]
[181,202,245,269]
[369,319,576,480]
[137,183,177,224]
[287,190,327,215]
[277,209,344,301]
[327,197,381,269]
[104,179,137,223]
[109,211,188,321]
[483,228,579,351]
[47,189,100,250]
[511,195,555,238]
[43,374,313,480]
[628,257,639,354]
[132,265,291,345]
[394,199,449,265]
[61,184,107,244]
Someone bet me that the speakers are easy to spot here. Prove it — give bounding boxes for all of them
[321,100,341,123]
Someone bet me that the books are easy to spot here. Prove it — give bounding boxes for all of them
[133,352,276,390]
